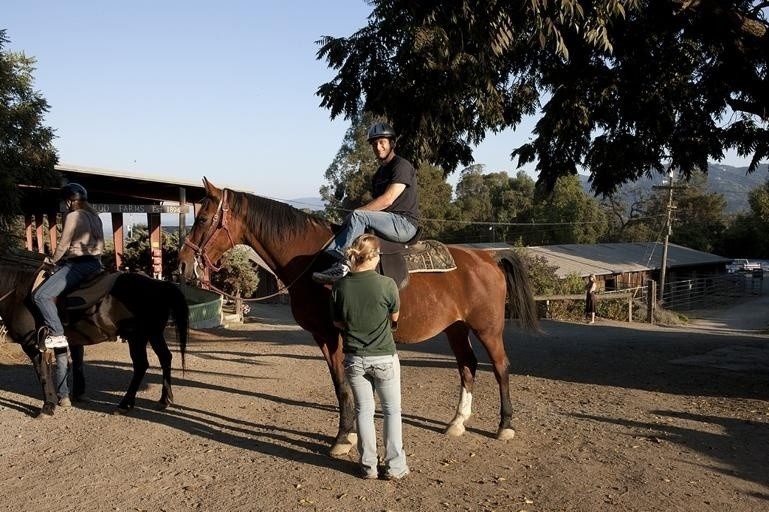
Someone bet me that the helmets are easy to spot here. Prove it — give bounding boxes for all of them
[368,122,396,142]
[59,183,88,202]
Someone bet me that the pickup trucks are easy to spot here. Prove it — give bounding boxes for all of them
[726,259,763,273]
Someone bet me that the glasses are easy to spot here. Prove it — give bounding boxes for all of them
[376,250,384,256]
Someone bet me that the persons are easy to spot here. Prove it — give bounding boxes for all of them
[328,233,409,480]
[50,347,85,406]
[312,122,418,282]
[585,273,597,324]
[32,182,105,350]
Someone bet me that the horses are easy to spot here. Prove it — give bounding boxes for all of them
[0,245,190,419]
[176,176,551,455]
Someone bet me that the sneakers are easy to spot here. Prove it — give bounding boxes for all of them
[363,465,409,480]
[312,263,350,283]
[35,335,70,349]
